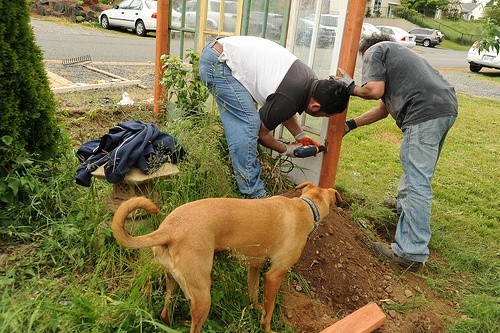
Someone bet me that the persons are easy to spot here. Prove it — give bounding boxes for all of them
[329,27,458,273]
[198,34,349,201]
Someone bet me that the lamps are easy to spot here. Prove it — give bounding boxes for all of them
[91,159,180,218]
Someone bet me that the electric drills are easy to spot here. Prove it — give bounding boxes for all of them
[284,140,326,158]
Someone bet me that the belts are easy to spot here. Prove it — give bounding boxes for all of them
[210,39,224,55]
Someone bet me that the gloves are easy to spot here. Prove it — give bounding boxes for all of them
[341,118,359,138]
[279,141,303,158]
[294,132,321,147]
[328,67,358,98]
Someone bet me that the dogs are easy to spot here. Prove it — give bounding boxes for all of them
[111,182,344,333]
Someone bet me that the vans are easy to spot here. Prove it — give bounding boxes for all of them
[176,0,237,31]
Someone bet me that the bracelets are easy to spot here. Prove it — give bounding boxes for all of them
[346,119,357,130]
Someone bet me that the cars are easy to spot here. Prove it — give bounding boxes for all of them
[98,0,181,36]
[249,10,338,48]
[466,35,500,72]
[360,22,416,48]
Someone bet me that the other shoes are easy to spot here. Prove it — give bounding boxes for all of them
[383,198,400,216]
[372,241,421,273]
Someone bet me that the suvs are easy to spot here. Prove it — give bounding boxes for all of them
[407,28,445,47]
